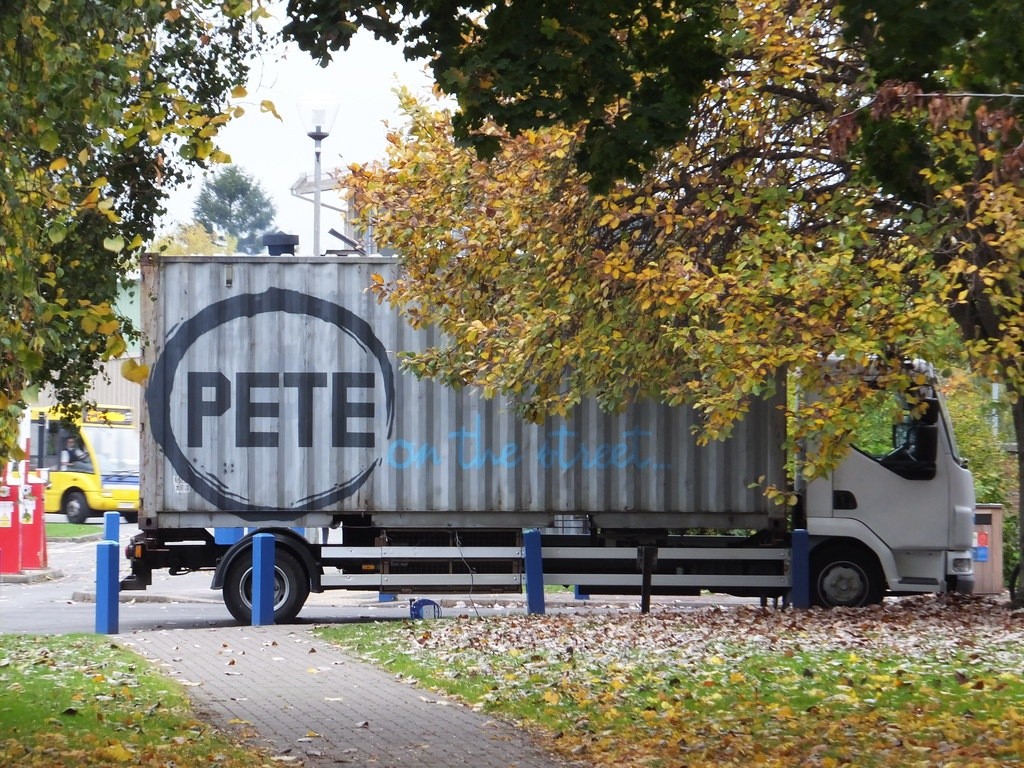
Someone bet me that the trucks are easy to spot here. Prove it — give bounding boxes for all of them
[119,252,975,624]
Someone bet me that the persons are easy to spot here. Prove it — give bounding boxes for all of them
[59,435,87,471]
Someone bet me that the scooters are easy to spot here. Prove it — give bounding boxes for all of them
[21,404,139,525]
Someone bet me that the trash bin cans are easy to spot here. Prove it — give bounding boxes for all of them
[973,504,1004,595]
